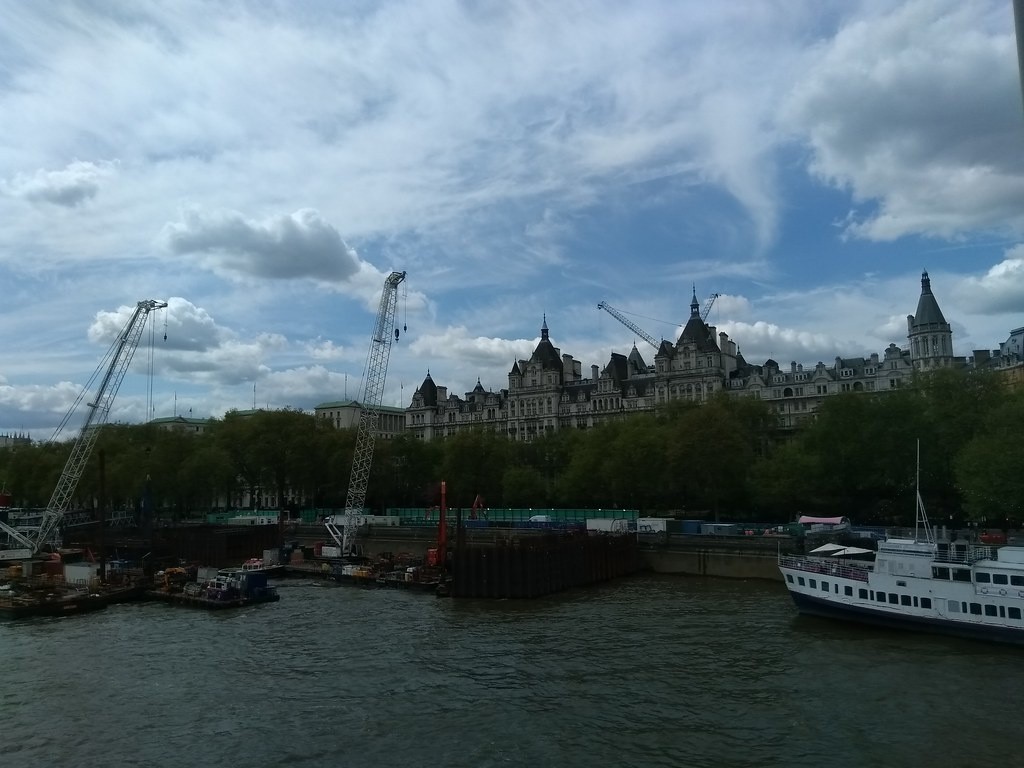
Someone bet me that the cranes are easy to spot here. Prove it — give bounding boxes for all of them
[596,291,722,350]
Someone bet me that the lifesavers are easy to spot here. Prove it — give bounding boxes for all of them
[999,589,1007,595]
[981,587,988,594]
[1018,590,1024,597]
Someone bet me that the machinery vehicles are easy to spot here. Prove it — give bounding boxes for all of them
[315,269,411,560]
[0,299,168,584]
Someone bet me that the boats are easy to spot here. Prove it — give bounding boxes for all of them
[777,438,1024,651]
